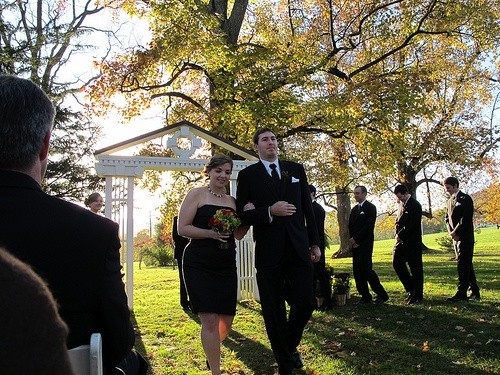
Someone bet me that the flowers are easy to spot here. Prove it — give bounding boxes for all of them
[209,208,242,250]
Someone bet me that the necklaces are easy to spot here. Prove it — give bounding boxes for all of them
[207,184,225,198]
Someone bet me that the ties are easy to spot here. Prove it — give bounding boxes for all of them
[269,163,280,191]
[357,205,361,212]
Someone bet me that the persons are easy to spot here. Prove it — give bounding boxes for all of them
[392,185,423,305]
[173,216,190,310]
[237,127,322,375]
[348,186,389,305]
[304,185,333,312]
[0,75,148,375]
[85,192,104,213]
[443,177,480,302]
[177,154,256,374]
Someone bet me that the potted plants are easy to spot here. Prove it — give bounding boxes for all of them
[316,278,350,307]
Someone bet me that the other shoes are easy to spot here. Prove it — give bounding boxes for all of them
[183,306,189,311]
[206,360,211,370]
[189,302,198,314]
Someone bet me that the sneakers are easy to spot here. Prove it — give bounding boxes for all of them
[405,291,414,302]
[406,292,423,306]
[352,294,374,308]
[467,291,480,300]
[447,292,469,303]
[292,347,303,370]
[317,303,332,312]
[374,293,389,305]
